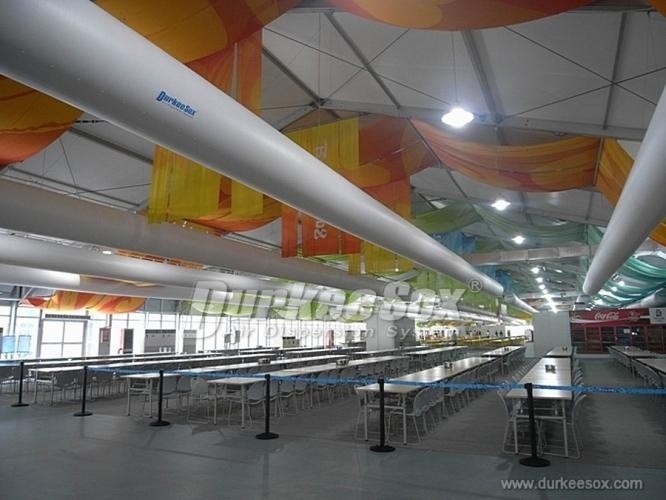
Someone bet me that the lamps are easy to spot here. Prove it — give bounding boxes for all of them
[440,30,558,313]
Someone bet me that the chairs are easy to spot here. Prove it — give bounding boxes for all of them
[1,337,665,461]
